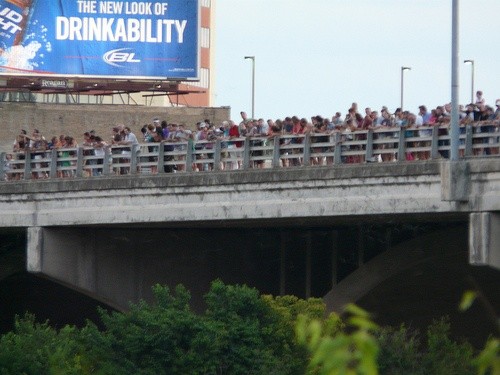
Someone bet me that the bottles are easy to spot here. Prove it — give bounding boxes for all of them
[0,0,34,57]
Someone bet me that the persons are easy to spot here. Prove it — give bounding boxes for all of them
[5,153,15,181]
[13,90,500,180]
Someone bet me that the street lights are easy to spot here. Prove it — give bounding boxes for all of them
[243,55,256,135]
[400,66,411,126]
[463,59,474,121]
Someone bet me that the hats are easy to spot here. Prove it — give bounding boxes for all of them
[459,105,468,112]
[200,123,206,128]
[153,118,160,123]
